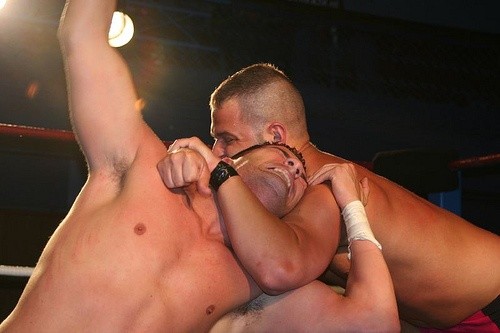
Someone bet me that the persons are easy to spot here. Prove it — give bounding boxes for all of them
[0,0,403,333]
[157,62,500,333]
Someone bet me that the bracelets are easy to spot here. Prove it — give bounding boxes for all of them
[208,161,239,190]
